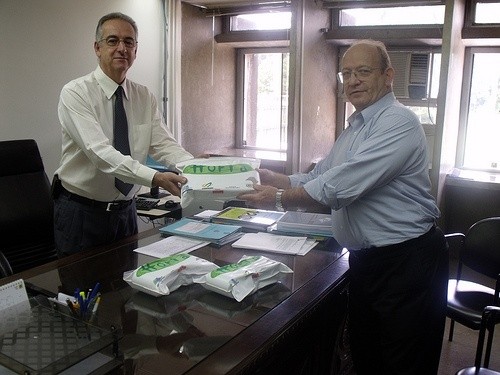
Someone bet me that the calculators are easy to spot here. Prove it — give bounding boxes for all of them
[137,199,160,211]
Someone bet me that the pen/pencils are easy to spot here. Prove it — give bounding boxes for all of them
[67,283,105,322]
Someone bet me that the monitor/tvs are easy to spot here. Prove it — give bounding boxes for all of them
[137,152,179,198]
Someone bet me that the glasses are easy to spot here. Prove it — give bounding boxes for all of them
[336,67,381,84]
[99,37,138,48]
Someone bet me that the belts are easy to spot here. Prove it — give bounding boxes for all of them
[61,187,134,212]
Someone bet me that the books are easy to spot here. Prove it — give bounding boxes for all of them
[231,231,320,256]
[159,218,244,248]
[209,207,286,233]
[277,211,336,237]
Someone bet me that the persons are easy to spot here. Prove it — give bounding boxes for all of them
[52,12,196,260]
[237,37,450,375]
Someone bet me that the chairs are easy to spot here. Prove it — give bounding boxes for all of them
[0,139,58,279]
[445,216,500,372]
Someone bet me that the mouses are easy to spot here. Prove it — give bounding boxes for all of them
[165,200,174,208]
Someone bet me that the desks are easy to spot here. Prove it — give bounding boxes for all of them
[0,209,352,375]
[133,188,182,218]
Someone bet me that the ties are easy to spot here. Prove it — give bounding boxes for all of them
[115,86,134,197]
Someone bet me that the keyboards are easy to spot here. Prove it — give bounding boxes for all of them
[136,199,160,210]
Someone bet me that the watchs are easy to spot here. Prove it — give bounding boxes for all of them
[275,189,285,212]
